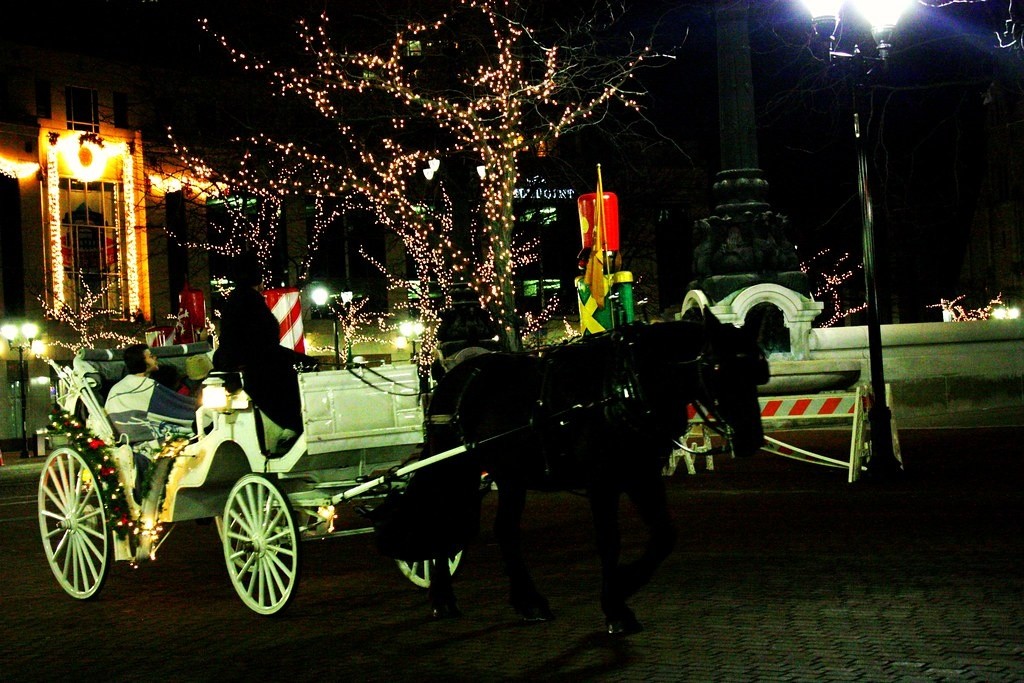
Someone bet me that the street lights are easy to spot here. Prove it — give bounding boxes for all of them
[778,0,936,488]
[1,320,45,457]
[396,322,426,364]
[310,284,341,370]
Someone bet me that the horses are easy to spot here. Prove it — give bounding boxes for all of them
[414,304,770,636]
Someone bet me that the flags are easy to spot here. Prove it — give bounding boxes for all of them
[584,181,604,308]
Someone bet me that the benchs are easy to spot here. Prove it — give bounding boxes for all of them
[88,415,145,471]
[205,368,244,408]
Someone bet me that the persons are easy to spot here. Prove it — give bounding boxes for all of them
[213,259,281,371]
[941,302,952,323]
[178,354,214,396]
[104,343,159,413]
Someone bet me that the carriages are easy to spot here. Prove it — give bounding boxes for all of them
[36,305,770,639]
